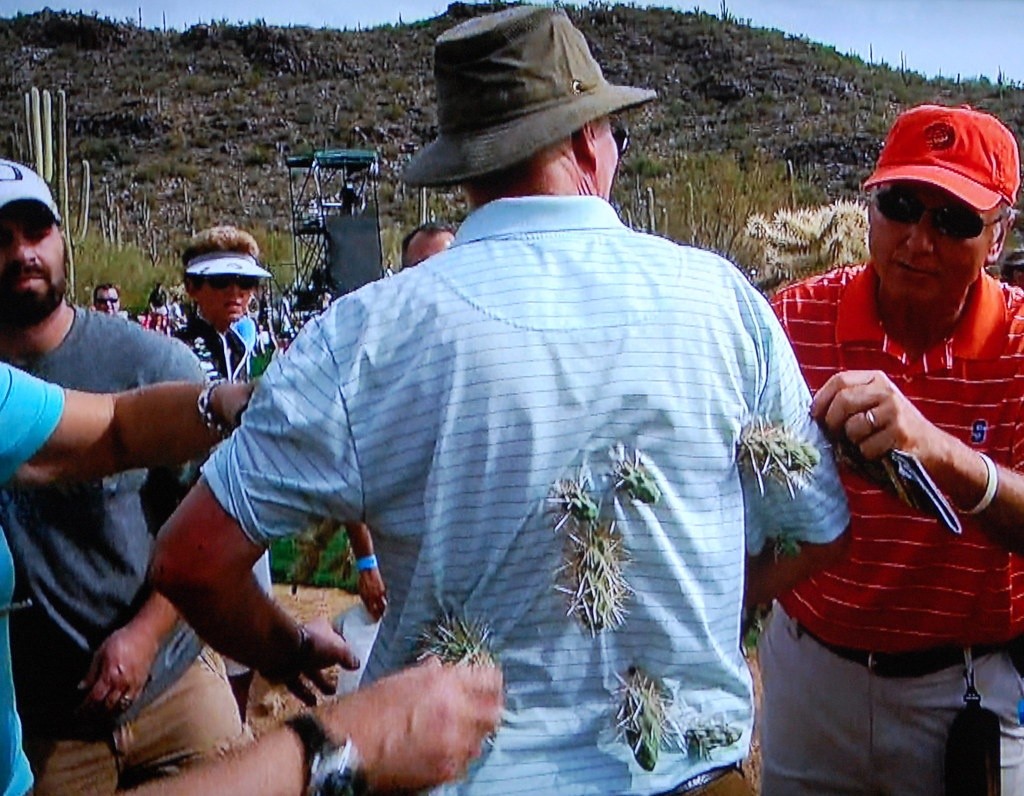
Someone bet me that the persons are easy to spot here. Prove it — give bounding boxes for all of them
[152,7,847,795]
[1,106,1024,795]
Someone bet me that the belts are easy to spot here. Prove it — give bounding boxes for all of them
[652,760,745,796]
[796,623,1011,679]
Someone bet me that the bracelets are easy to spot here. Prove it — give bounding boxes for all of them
[357,556,377,574]
[195,377,234,440]
[948,452,997,514]
[271,623,308,681]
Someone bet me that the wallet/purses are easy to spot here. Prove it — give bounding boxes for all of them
[945,668,1001,796]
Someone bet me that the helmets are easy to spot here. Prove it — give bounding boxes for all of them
[0,158,61,226]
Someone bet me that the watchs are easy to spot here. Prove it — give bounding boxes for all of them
[282,715,364,795]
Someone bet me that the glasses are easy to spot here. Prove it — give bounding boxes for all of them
[610,117,629,154]
[875,184,1002,238]
[0,216,53,245]
[96,299,117,303]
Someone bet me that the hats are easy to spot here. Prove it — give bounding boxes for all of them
[186,250,272,278]
[863,105,1021,212]
[401,6,657,187]
[204,275,257,290]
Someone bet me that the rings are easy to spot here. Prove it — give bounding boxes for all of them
[119,696,133,710]
[866,410,880,435]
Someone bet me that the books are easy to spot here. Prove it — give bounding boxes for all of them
[891,448,962,532]
